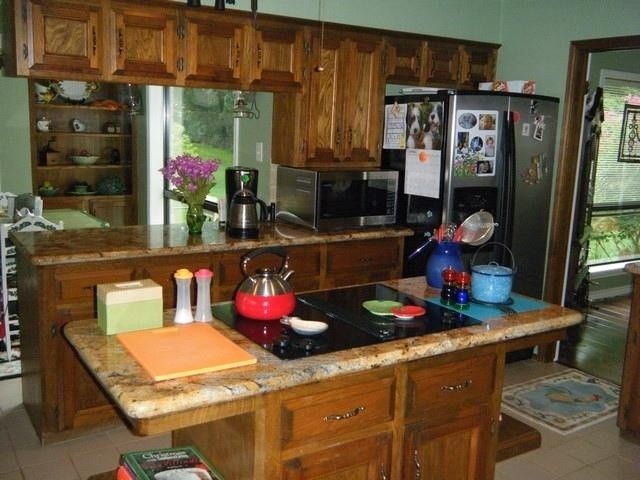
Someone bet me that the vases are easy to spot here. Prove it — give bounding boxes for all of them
[186,203,206,236]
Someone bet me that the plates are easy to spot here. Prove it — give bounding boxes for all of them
[67,191,96,195]
[469,296,513,308]
[390,305,425,321]
[291,320,330,335]
[361,299,401,316]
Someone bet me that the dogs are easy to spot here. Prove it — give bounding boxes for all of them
[424,101,444,151]
[405,103,427,150]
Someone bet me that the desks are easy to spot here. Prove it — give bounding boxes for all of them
[61,271,587,479]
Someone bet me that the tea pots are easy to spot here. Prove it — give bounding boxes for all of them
[235,246,298,320]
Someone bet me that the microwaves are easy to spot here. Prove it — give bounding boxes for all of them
[277,163,401,230]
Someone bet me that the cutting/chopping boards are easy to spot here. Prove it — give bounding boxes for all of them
[115,323,258,382]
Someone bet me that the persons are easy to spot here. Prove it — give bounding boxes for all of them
[485,137,494,157]
[455,132,468,164]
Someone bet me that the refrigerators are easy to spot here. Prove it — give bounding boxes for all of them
[381,94,558,363]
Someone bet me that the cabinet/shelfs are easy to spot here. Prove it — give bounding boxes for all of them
[180,2,247,91]
[108,0,178,86]
[422,33,460,89]
[191,215,324,308]
[5,220,212,449]
[272,22,385,172]
[461,37,499,91]
[385,29,421,88]
[249,8,302,93]
[26,73,140,230]
[0,1,107,80]
[315,225,418,292]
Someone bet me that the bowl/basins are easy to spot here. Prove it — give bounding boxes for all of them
[39,186,61,196]
[67,155,100,164]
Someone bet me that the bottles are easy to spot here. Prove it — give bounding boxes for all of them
[104,119,121,133]
[196,269,213,322]
[173,268,194,323]
[426,243,464,289]
[441,268,455,305]
[37,117,50,131]
[454,271,470,305]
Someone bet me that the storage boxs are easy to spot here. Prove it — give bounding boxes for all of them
[95,276,167,337]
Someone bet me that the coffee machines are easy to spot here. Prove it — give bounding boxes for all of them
[224,166,267,238]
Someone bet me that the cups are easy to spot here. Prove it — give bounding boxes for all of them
[73,186,87,192]
[478,82,491,92]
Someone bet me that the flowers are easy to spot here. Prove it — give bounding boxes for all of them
[156,148,227,235]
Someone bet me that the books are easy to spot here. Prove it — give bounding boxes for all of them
[116,444,226,480]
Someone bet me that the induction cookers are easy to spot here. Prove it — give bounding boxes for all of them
[206,285,482,363]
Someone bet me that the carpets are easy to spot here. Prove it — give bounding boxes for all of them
[499,366,622,437]
